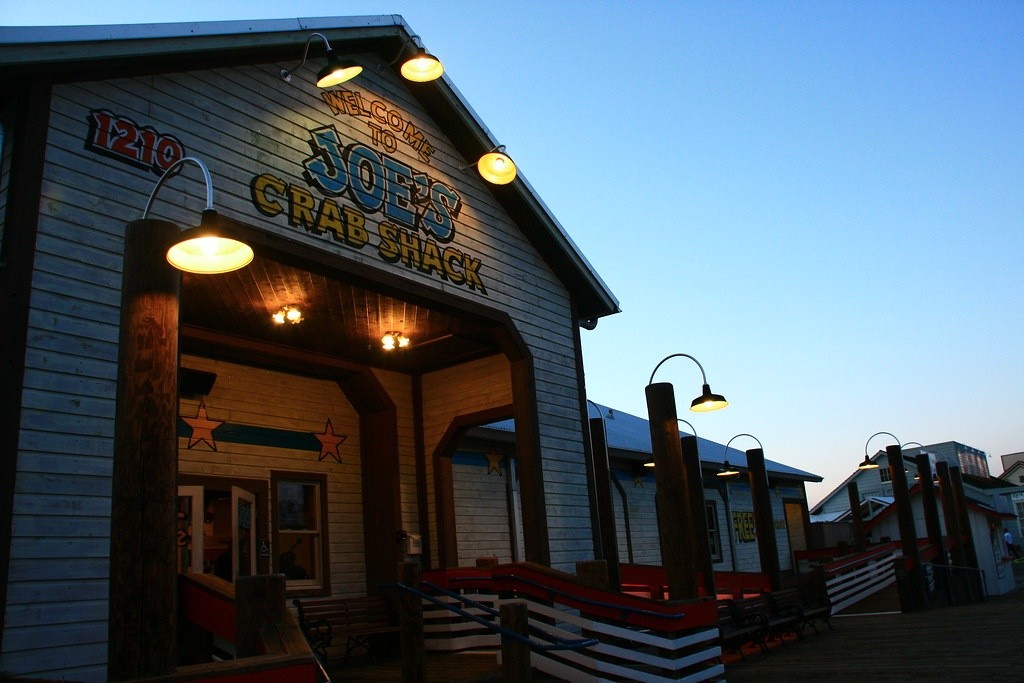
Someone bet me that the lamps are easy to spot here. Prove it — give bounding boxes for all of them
[263,302,312,331]
[457,143,518,188]
[381,35,454,84]
[277,33,365,90]
[374,331,416,356]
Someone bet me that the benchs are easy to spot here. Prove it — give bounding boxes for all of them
[291,595,400,669]
[703,588,833,663]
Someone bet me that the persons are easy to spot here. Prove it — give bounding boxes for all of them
[215,548,233,583]
[1004,529,1019,556]
